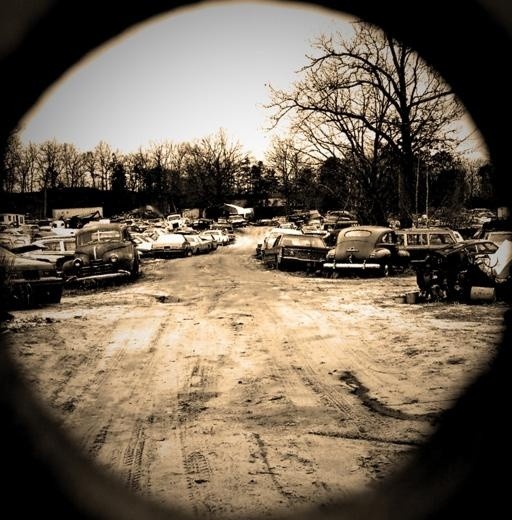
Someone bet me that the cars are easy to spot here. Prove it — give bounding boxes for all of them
[227,216,246,227]
[0,212,233,309]
[259,207,498,276]
